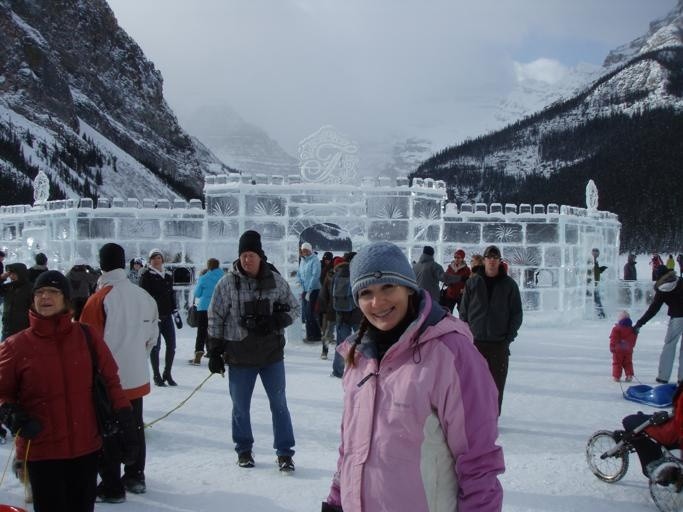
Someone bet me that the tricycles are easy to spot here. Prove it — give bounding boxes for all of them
[583,410,681,512]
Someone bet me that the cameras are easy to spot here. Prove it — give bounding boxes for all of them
[241,315,259,330]
[273,301,291,313]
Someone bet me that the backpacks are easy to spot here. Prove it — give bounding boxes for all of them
[333,267,354,313]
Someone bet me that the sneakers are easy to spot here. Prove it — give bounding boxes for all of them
[302,331,341,379]
[279,455,296,471]
[240,448,255,467]
[613,372,668,384]
[121,472,146,493]
[94,482,126,502]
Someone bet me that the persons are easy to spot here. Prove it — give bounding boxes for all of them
[590,247,683,384]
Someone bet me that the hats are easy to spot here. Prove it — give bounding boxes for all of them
[133,257,142,266]
[32,272,67,295]
[97,243,126,270]
[652,265,670,279]
[147,248,164,264]
[350,242,420,306]
[300,243,357,265]
[238,231,262,255]
[423,246,502,257]
[35,253,47,266]
[592,248,682,263]
[616,309,631,318]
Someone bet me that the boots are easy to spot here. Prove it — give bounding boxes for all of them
[163,350,177,385]
[151,344,165,386]
[186,350,204,367]
[204,345,211,357]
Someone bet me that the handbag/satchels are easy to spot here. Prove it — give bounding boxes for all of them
[92,377,122,446]
[187,305,199,327]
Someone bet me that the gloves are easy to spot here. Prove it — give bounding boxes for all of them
[296,282,301,288]
[175,311,183,329]
[305,293,310,301]
[9,409,40,439]
[251,317,272,337]
[120,408,140,438]
[209,352,227,374]
